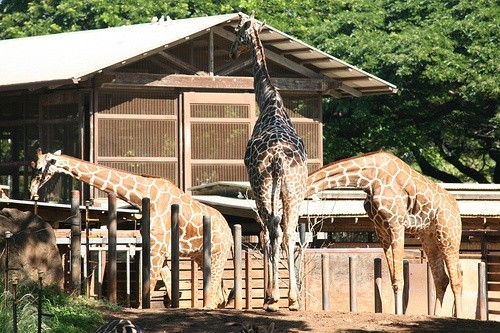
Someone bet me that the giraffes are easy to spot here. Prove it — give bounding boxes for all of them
[228,9,308,312]
[28,144,235,309]
[257,149,463,318]
